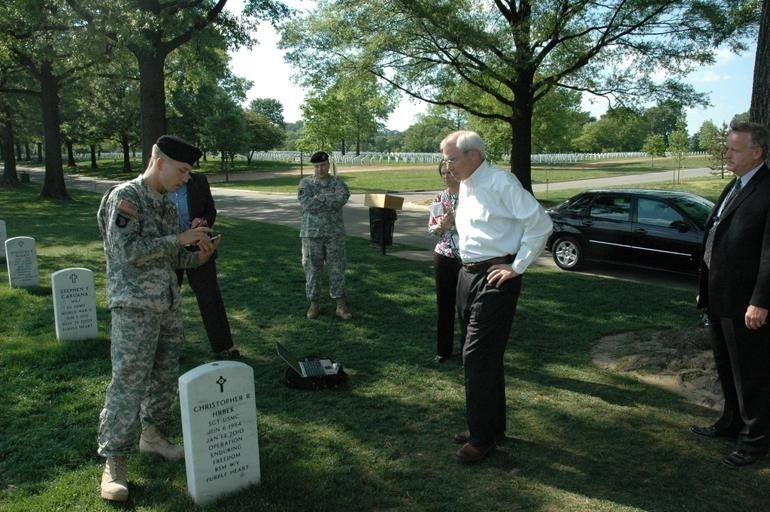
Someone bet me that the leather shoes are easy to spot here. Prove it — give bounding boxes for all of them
[453,430,505,444]
[215,350,240,360]
[456,440,496,461]
[690,421,738,439]
[721,448,768,469]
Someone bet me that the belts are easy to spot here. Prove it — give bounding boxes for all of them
[463,255,515,272]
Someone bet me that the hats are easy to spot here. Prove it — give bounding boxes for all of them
[311,152,328,162]
[156,135,202,166]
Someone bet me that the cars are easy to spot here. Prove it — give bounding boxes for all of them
[543,187,716,282]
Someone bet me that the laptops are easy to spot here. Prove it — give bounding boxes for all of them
[275,341,337,379]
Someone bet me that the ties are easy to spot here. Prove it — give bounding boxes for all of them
[703,178,741,270]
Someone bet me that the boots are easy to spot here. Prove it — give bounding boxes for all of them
[100,456,129,502]
[335,298,352,320]
[139,424,184,459]
[307,300,320,318]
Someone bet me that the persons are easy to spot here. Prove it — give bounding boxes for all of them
[297,150,353,321]
[693,118,769,467]
[427,157,463,364]
[166,171,241,362]
[440,129,554,461]
[96,133,224,501]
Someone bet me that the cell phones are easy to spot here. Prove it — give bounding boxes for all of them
[197,233,221,245]
